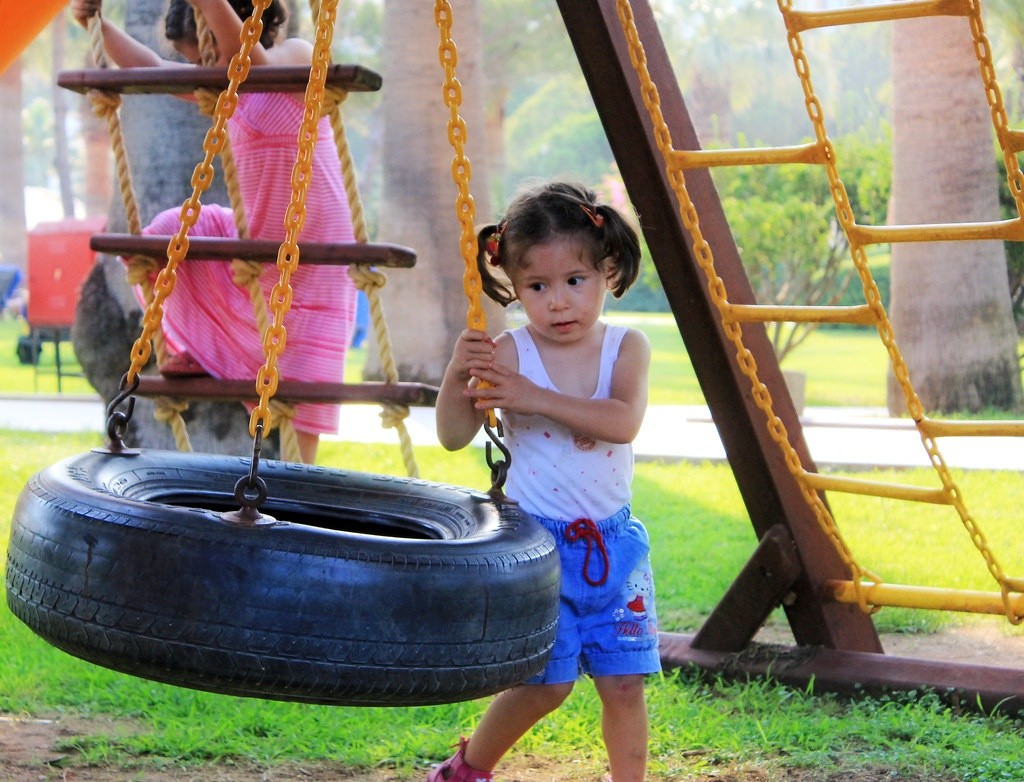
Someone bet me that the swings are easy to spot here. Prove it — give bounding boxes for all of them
[5,1,561,708]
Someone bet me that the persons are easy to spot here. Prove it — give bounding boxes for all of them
[68,1,360,472]
[437,175,664,782]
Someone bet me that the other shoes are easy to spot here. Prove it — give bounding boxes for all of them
[427,736,493,782]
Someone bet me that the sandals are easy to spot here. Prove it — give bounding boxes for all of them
[159,350,208,376]
